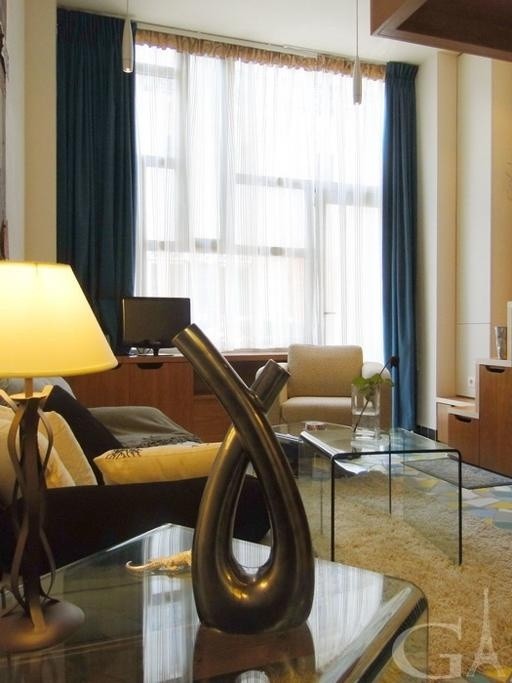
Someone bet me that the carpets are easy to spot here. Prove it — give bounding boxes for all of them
[401,459,512,489]
[85,470,512,683]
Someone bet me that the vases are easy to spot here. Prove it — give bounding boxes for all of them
[350,385,392,431]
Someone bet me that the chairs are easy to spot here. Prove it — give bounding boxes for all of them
[253,343,391,426]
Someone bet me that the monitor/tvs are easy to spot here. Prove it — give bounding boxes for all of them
[121,296,191,356]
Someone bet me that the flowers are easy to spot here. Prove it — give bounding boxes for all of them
[351,355,399,431]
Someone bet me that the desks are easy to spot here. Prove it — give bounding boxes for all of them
[0,521,429,683]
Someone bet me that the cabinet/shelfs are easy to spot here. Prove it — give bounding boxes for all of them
[65,350,287,443]
[436,359,512,477]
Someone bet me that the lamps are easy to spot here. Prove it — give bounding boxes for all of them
[0,260,120,655]
[353,0,362,105]
[122,0,134,73]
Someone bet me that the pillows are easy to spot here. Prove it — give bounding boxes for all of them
[41,385,123,485]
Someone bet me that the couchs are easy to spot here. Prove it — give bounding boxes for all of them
[0,376,270,576]
